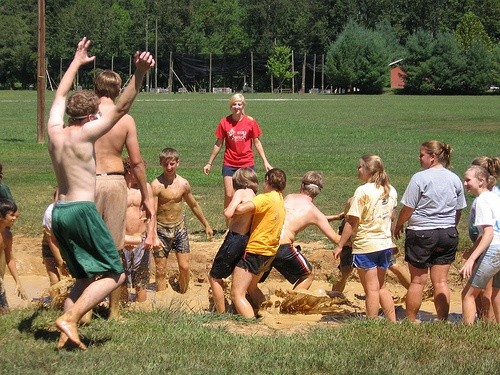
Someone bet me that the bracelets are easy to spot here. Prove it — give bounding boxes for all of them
[208,162,212,166]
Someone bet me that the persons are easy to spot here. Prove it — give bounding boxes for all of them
[459,156,500,326]
[394,140,467,324]
[0,198,28,314]
[151,148,213,294]
[204,93,273,228]
[78,70,153,326]
[208,168,259,313]
[42,191,71,301]
[0,162,14,202]
[332,155,397,324]
[257,171,352,293]
[119,156,154,303]
[48,37,155,351]
[223,168,286,320]
[332,197,410,292]
